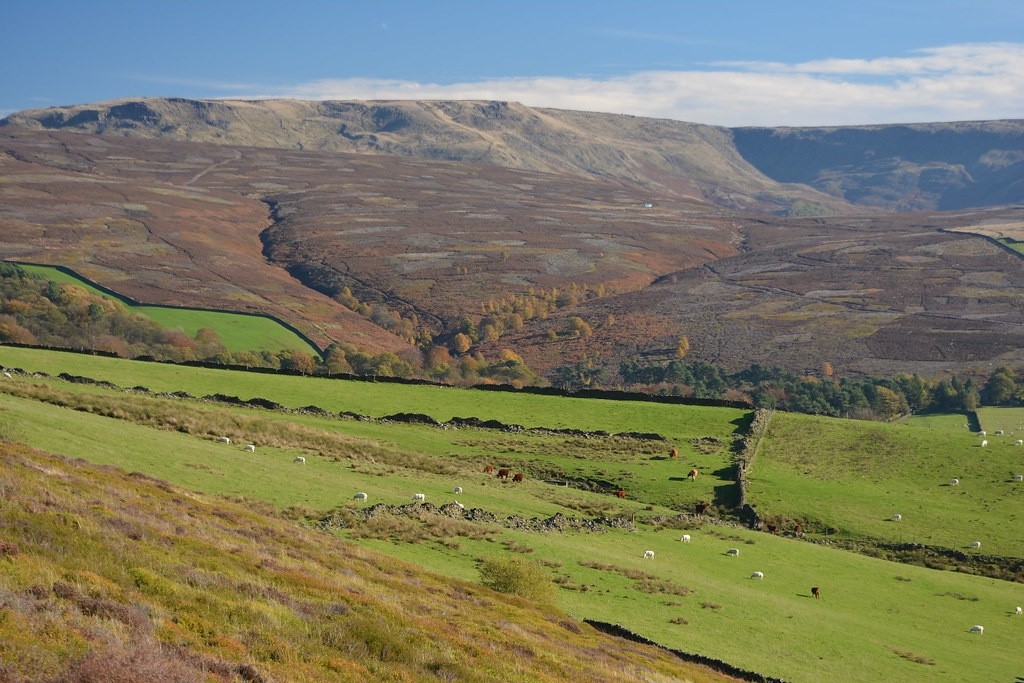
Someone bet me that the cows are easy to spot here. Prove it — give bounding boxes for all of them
[484,465,524,483]
[696,501,707,517]
[794,526,801,533]
[767,524,779,535]
[669,448,698,481]
[811,588,821,598]
[614,491,626,500]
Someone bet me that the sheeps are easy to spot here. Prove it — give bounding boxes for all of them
[750,571,763,580]
[969,625,984,635]
[243,445,256,453]
[292,456,305,465]
[969,541,981,549]
[726,548,740,557]
[891,514,901,520]
[643,550,655,559]
[679,535,690,543]
[1012,474,1023,483]
[352,492,368,501]
[216,437,230,445]
[950,478,960,487]
[973,429,1024,449]
[411,493,425,503]
[453,486,465,509]
[1014,606,1023,615]
